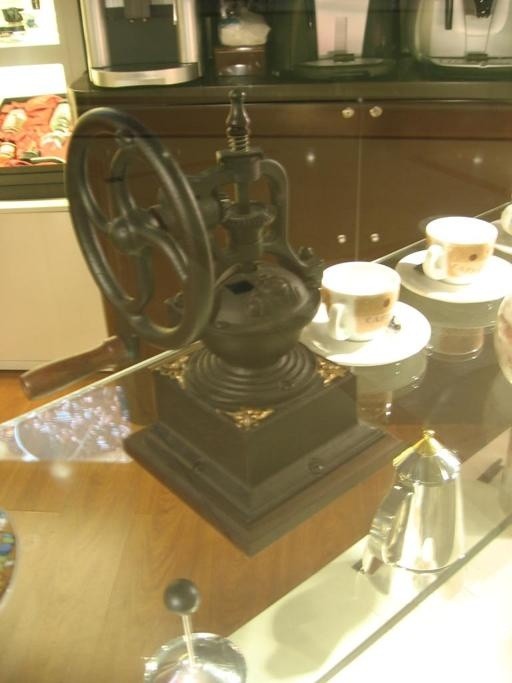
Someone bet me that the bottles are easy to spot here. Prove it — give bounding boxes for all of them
[3,99,70,139]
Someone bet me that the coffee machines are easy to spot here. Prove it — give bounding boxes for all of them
[267,1,512,82]
[78,1,207,88]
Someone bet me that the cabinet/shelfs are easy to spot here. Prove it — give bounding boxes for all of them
[69,69,512,337]
[1,196,109,371]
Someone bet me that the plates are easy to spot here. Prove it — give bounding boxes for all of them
[395,249,512,306]
[304,295,430,367]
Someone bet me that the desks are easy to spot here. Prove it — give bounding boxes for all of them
[1,198,512,683]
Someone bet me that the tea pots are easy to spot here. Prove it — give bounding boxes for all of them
[368,428,468,575]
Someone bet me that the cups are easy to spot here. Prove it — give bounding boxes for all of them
[419,216,497,284]
[316,262,400,340]
